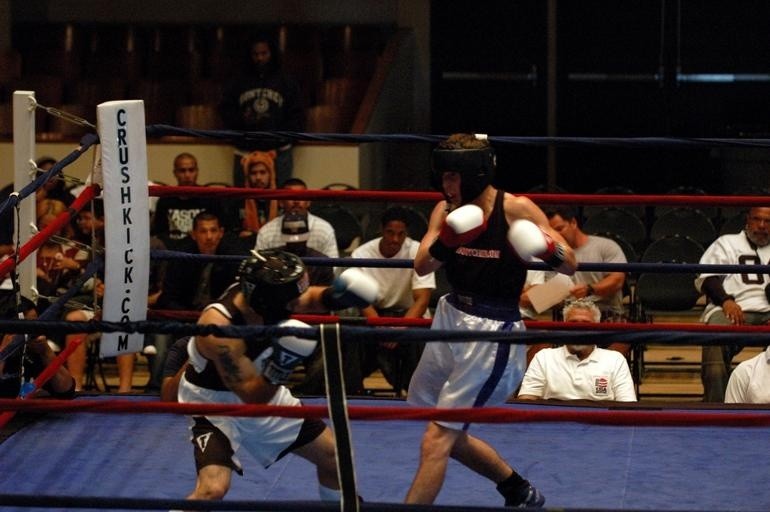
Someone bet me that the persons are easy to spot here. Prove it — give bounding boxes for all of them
[175,249,365,501]
[402,132,579,509]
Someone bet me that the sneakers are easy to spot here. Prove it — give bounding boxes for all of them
[514,482,545,508]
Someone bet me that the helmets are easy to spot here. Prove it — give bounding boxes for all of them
[237,249,310,319]
[429,149,499,201]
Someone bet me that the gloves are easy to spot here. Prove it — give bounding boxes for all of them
[438,205,489,251]
[505,219,556,264]
[320,265,379,312]
[264,319,318,385]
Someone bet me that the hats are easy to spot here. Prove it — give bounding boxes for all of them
[282,212,311,244]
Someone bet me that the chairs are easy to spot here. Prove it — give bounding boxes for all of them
[1,7,769,420]
